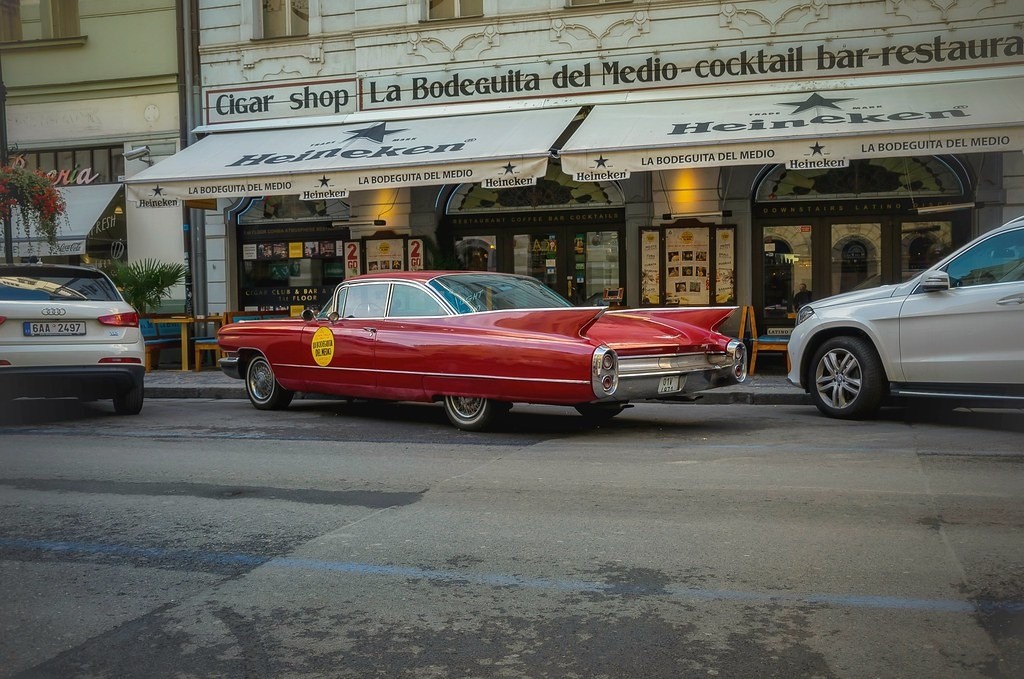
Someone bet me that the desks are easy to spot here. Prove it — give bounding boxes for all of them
[149,316,223,372]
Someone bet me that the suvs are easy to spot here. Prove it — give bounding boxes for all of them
[784,212,1024,417]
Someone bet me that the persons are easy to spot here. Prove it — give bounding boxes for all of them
[794,283,816,313]
[765,284,789,313]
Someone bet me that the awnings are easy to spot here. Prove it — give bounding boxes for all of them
[0,184,122,258]
[557,74,1024,183]
[123,104,582,203]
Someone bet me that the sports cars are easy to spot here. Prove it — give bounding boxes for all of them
[216,269,749,431]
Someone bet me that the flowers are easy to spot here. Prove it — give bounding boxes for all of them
[0,163,76,267]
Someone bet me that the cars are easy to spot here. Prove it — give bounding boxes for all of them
[0,263,146,418]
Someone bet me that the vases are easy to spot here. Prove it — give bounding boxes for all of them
[15,187,26,207]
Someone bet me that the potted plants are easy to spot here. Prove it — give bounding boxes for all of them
[99,255,191,370]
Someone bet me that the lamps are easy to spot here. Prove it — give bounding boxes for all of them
[663,209,734,220]
[906,198,986,217]
[321,219,387,228]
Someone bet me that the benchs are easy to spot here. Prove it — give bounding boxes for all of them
[727,305,792,377]
[138,313,217,373]
[194,310,292,372]
